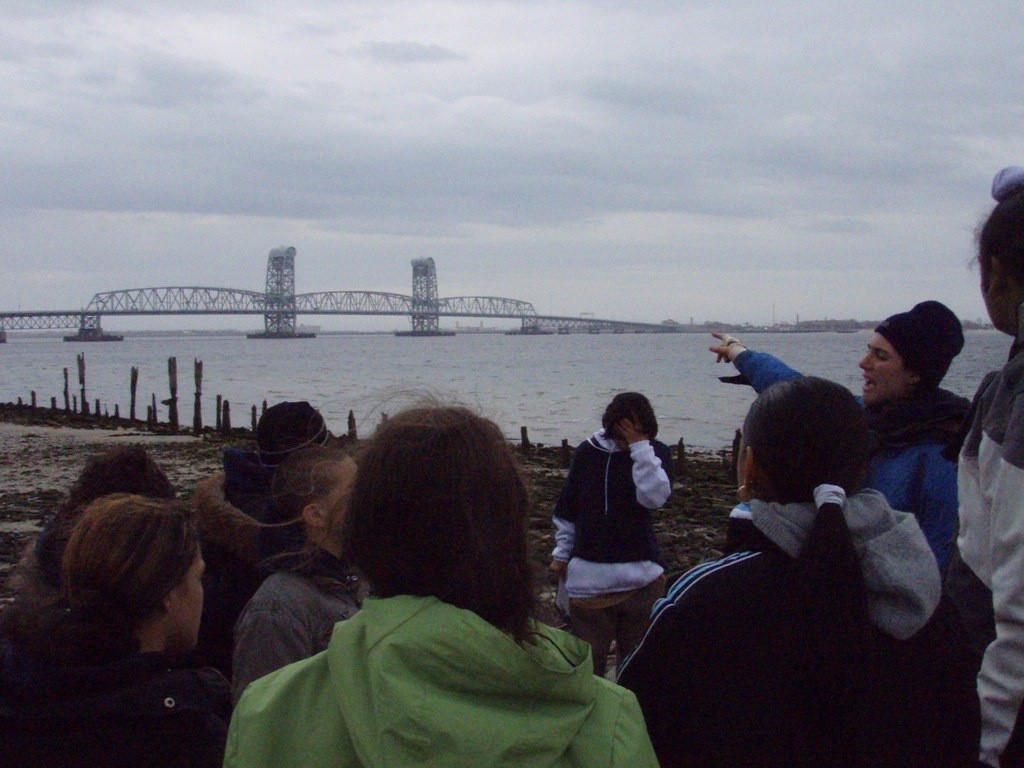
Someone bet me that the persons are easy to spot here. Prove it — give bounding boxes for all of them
[547,393,670,677]
[224,406,662,767]
[0,401,363,768]
[616,165,1024,768]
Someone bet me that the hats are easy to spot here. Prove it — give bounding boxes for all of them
[257,401,331,471]
[874,299,964,394]
